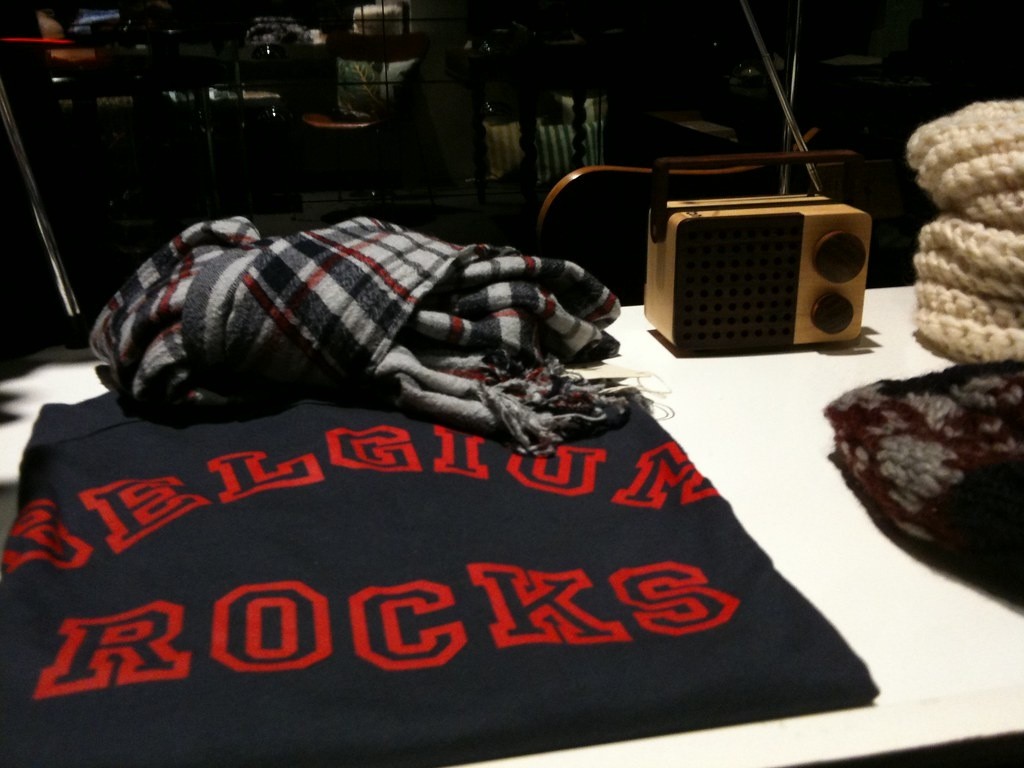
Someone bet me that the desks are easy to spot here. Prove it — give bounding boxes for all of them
[0,286,1024,768]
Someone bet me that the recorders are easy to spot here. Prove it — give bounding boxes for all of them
[649,0,871,353]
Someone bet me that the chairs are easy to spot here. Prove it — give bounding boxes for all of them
[294,30,440,224]
[534,148,866,307]
[31,9,137,167]
